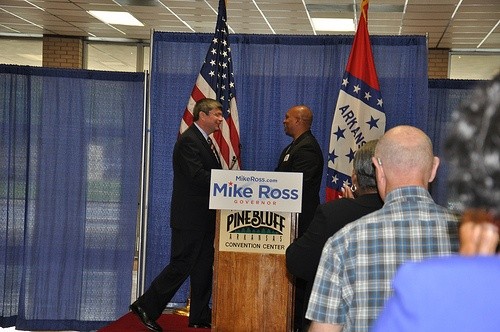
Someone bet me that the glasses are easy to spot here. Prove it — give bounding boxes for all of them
[206,111,224,118]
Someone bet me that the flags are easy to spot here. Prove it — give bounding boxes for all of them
[325,0,389,202]
[178,0,241,170]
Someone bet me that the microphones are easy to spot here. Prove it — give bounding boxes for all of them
[230,143,242,170]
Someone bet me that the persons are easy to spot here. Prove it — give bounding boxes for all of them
[128,97,225,332]
[275,105,324,237]
[285,74,500,332]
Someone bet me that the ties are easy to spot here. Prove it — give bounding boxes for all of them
[206,137,220,165]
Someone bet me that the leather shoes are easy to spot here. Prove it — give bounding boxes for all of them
[188,319,212,329]
[128,299,163,331]
